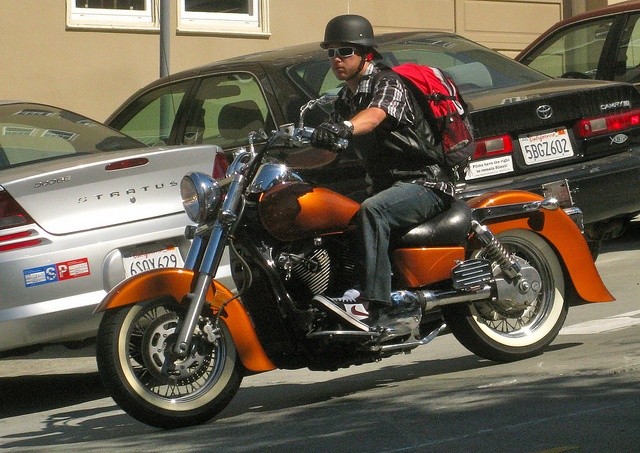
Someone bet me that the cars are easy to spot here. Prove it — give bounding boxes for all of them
[104,30,640,239]
[0,98,236,358]
[514,0,640,89]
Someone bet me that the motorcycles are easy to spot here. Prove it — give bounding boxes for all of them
[92,92,616,427]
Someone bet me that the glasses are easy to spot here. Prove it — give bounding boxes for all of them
[325,48,363,59]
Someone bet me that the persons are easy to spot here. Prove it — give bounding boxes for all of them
[311,15,458,334]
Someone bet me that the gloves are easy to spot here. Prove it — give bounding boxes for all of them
[311,120,354,151]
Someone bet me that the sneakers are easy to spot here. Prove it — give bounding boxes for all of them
[312,288,374,331]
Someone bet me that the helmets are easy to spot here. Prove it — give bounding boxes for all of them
[321,15,378,47]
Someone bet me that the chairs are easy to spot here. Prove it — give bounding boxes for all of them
[217,100,265,139]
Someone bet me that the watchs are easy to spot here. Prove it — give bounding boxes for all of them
[341,120,355,135]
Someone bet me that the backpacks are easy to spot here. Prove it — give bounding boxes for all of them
[371,64,475,171]
